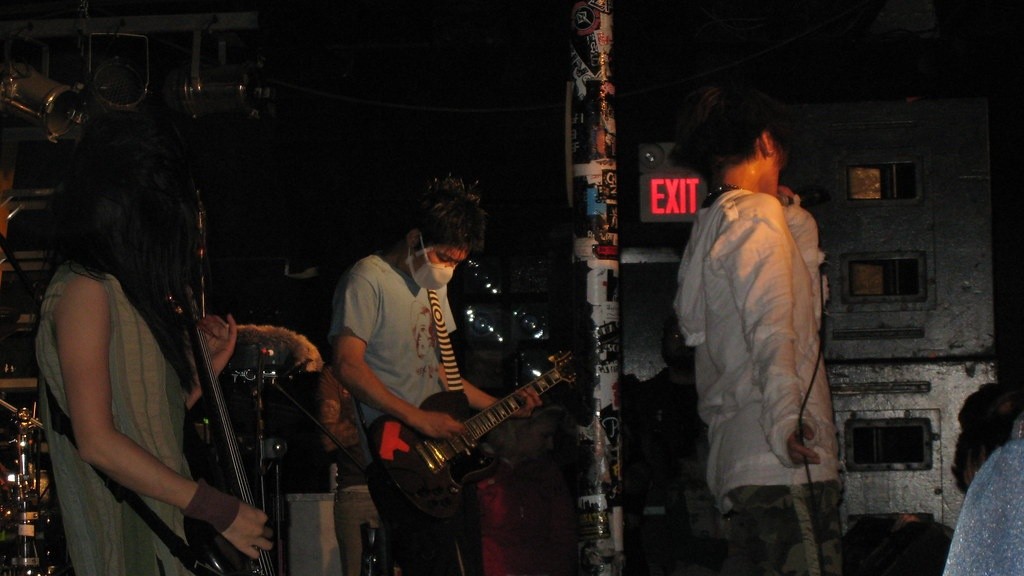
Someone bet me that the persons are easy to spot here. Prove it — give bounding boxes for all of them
[326,177,542,576]
[476,399,579,576]
[34,112,273,576]
[672,86,845,576]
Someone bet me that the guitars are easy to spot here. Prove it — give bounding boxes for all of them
[173,284,289,575]
[367,347,582,519]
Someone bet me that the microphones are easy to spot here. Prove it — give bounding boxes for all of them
[777,194,795,206]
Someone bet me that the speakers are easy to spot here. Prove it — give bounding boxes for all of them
[840,515,953,576]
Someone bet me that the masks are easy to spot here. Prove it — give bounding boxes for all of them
[407,233,458,291]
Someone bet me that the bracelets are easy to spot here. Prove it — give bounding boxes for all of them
[179,477,239,534]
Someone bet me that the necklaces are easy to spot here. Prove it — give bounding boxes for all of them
[705,184,740,199]
